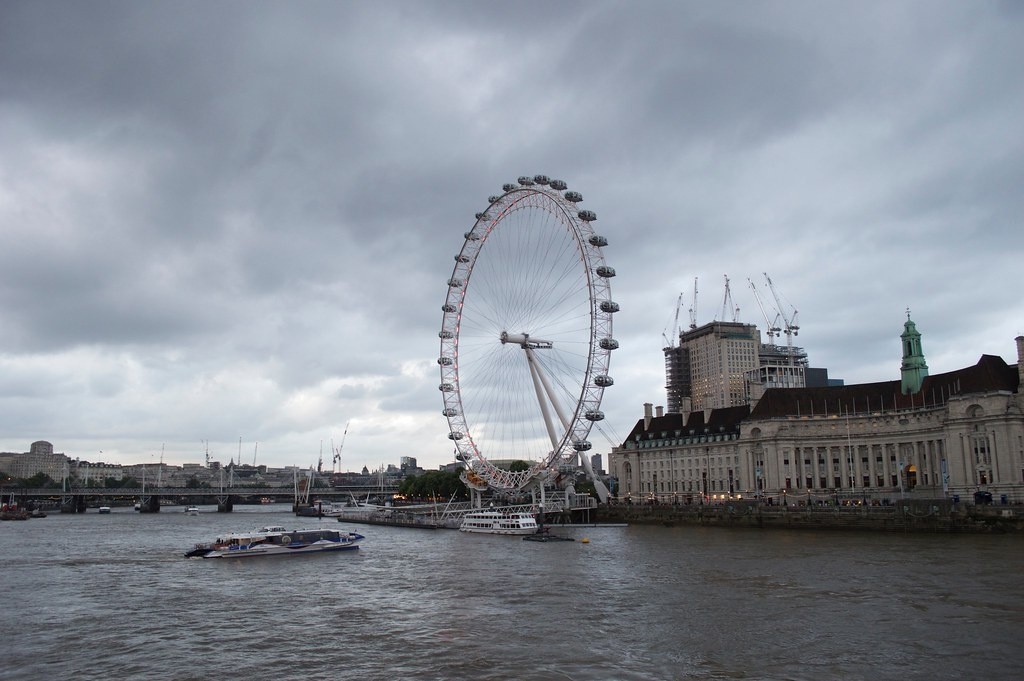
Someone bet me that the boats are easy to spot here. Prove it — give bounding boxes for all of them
[185,505,199,515]
[312,500,344,518]
[134,502,142,512]
[204,525,365,558]
[98,506,111,514]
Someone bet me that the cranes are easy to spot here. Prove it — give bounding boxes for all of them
[662,270,800,367]
[317,422,350,475]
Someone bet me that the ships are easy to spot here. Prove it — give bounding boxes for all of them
[458,512,539,536]
